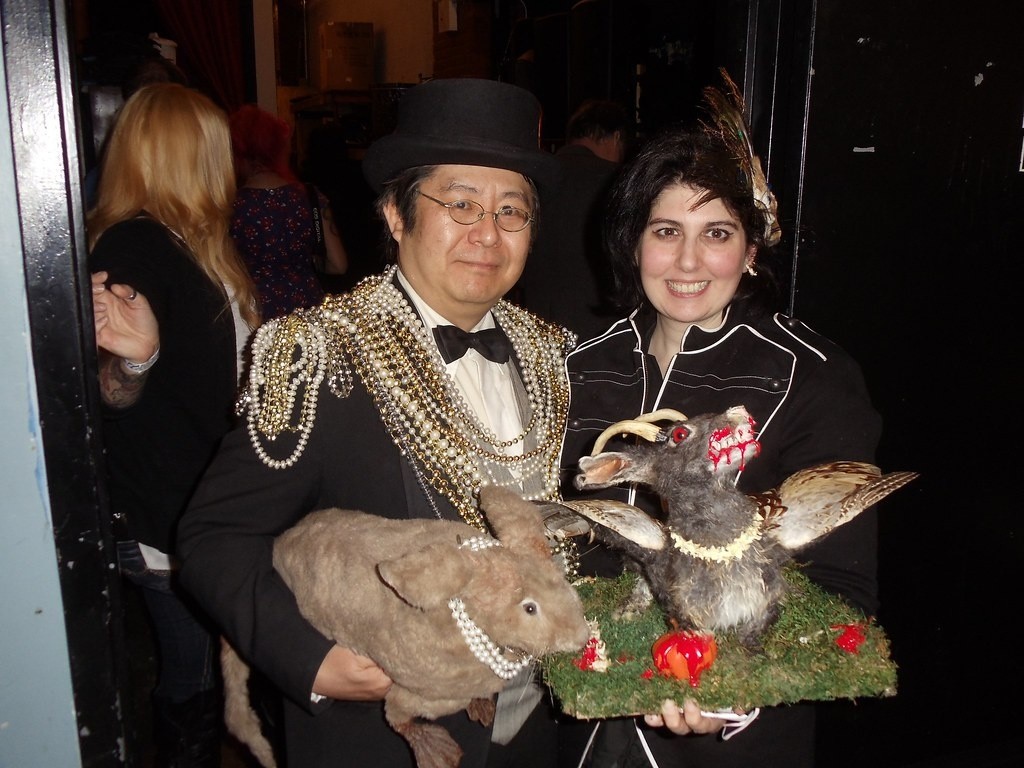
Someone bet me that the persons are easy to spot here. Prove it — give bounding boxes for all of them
[558,68,890,768]
[177,76,590,768]
[81,84,347,768]
[511,106,626,322]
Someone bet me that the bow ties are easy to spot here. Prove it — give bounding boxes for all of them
[432,323,511,366]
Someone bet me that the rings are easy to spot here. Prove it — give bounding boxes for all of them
[127,289,138,300]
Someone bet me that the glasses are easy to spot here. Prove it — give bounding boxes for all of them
[413,189,535,232]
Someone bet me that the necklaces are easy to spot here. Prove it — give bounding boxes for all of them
[452,537,535,679]
[238,263,578,531]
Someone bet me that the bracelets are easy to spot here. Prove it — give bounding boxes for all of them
[125,345,162,374]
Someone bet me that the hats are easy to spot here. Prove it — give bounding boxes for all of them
[381,77,545,179]
